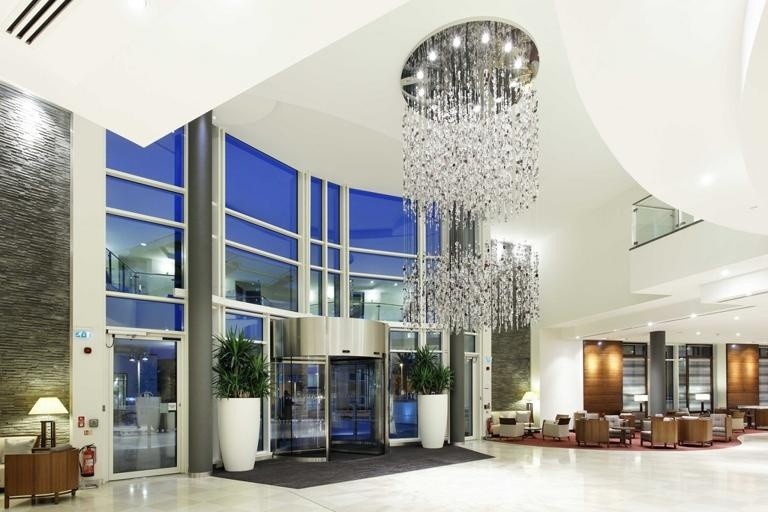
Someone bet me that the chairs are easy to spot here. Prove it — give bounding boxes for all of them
[0,433,41,487]
[490,403,768,449]
[3,446,82,509]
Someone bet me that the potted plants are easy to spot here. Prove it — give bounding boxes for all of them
[208,323,272,472]
[397,345,455,451]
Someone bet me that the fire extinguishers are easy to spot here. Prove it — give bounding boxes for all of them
[78,442,96,477]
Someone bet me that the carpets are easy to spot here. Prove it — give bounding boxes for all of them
[208,444,497,489]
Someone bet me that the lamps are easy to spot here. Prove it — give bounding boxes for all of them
[633,394,649,411]
[392,19,543,231]
[398,238,542,337]
[694,393,711,412]
[28,395,70,446]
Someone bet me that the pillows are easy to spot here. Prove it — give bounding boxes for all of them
[1,438,35,463]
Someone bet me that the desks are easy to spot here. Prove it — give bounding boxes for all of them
[31,447,49,455]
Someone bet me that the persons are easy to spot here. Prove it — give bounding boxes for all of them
[277,390,297,447]
